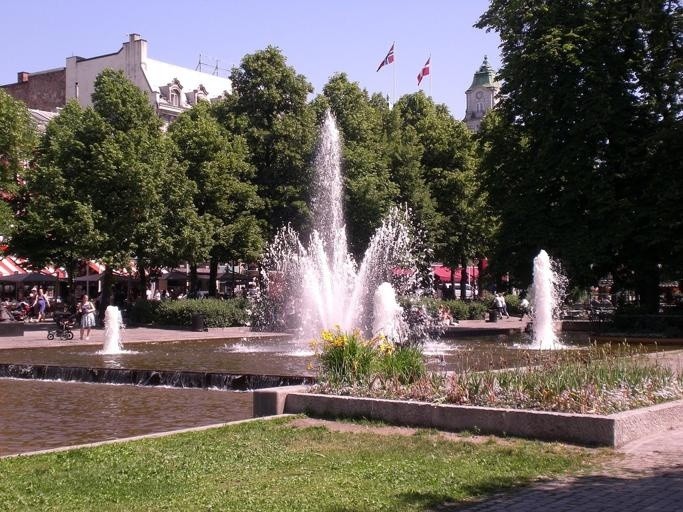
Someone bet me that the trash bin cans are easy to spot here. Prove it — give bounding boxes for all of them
[191,314,203,332]
[489,309,497,322]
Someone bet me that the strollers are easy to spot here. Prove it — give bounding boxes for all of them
[9,302,32,324]
[47,311,76,340]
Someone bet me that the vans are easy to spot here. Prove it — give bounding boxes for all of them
[444,281,478,299]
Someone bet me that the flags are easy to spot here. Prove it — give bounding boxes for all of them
[375,44,394,73]
[416,58,430,87]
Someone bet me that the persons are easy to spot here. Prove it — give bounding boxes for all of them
[489,294,502,320]
[435,304,444,321]
[518,297,533,321]
[76,295,96,341]
[442,306,454,325]
[498,292,510,319]
[25,287,38,319]
[30,288,51,323]
